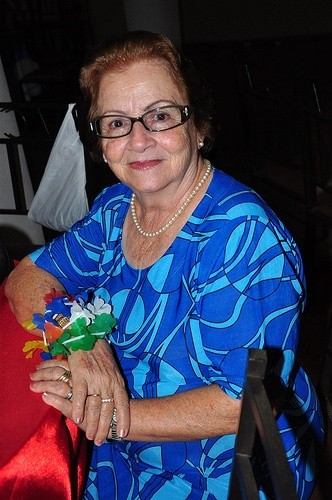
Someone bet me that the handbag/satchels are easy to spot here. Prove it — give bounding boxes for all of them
[28,103,90,233]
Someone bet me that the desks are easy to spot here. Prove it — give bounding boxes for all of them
[0,259,92,500]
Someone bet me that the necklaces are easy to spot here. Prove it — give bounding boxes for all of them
[130,158,212,237]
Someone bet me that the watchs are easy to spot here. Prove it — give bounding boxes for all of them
[111,407,122,440]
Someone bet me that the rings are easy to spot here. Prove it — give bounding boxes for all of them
[66,391,74,400]
[59,370,72,382]
[102,398,114,403]
[87,393,101,397]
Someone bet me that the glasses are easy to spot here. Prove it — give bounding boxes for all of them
[90,105,193,139]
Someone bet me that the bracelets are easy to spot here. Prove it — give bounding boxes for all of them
[22,286,116,361]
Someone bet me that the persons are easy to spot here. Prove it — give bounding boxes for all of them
[3,33,323,500]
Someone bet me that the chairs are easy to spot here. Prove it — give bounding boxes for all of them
[0,52,332,310]
[228,349,332,500]
